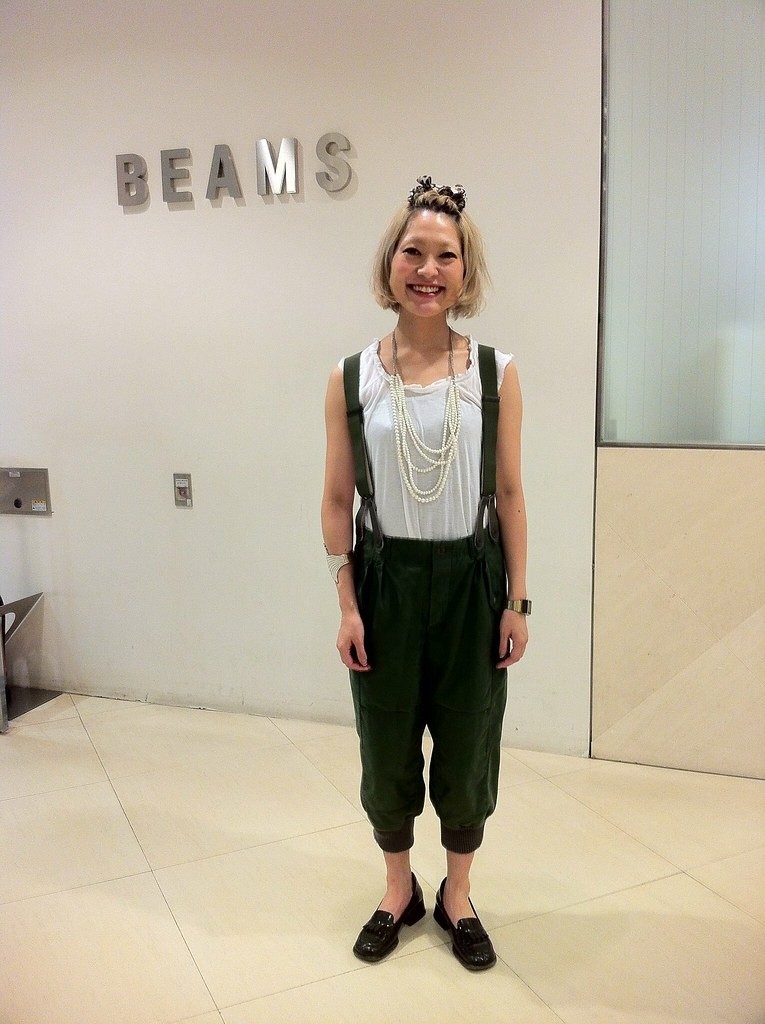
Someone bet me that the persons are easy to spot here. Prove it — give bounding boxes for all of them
[323,190,528,972]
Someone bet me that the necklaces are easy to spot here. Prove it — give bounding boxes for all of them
[391,326,461,503]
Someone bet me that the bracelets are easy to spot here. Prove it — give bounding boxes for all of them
[324,544,353,583]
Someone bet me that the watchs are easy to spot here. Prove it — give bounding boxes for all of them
[508,599,532,615]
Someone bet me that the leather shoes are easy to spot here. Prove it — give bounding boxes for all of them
[434,877,497,971]
[353,871,428,964]
